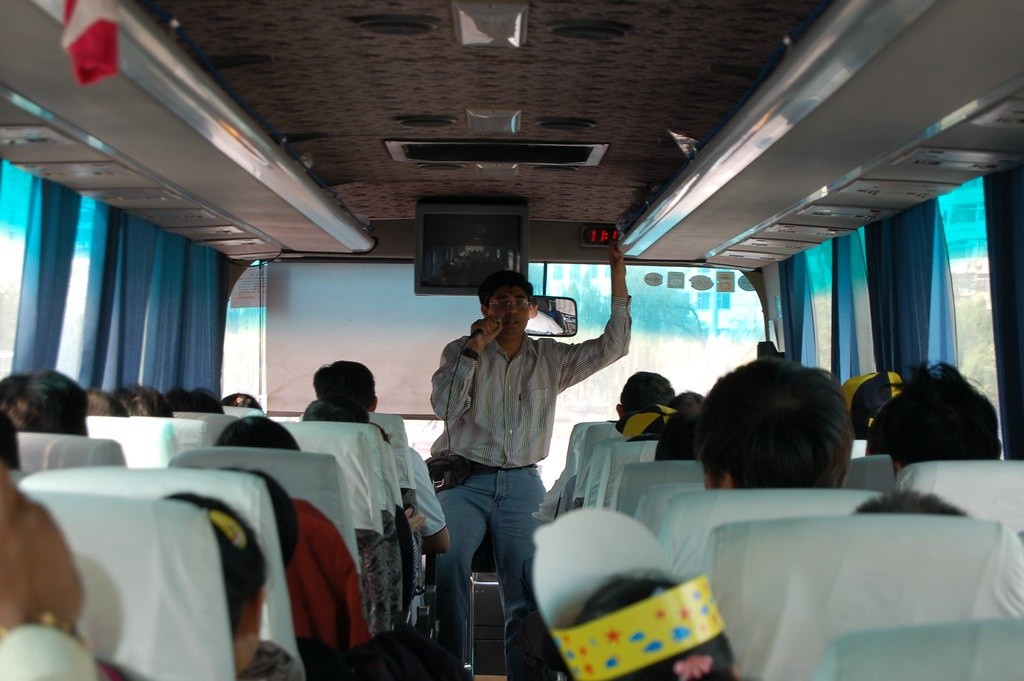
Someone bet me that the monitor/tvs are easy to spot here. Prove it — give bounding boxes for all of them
[414,202,529,296]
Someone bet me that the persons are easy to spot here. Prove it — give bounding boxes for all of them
[0,371,369,681]
[524,301,563,336]
[532,355,1003,681]
[425,235,632,681]
[312,361,450,555]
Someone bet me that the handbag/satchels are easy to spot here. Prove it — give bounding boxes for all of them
[424,455,468,493]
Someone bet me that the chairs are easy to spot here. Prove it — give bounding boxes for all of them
[0,403,441,681]
[532,420,1024,681]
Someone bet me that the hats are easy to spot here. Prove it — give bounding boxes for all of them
[842,371,903,416]
[165,493,266,603]
[616,403,678,441]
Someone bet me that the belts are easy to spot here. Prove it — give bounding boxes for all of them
[470,464,537,474]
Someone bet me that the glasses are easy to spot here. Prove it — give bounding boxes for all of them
[488,299,531,309]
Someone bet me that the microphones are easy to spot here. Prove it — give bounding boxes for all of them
[467,314,503,340]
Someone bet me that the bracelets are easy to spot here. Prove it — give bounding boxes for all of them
[28,613,91,648]
[463,348,479,362]
